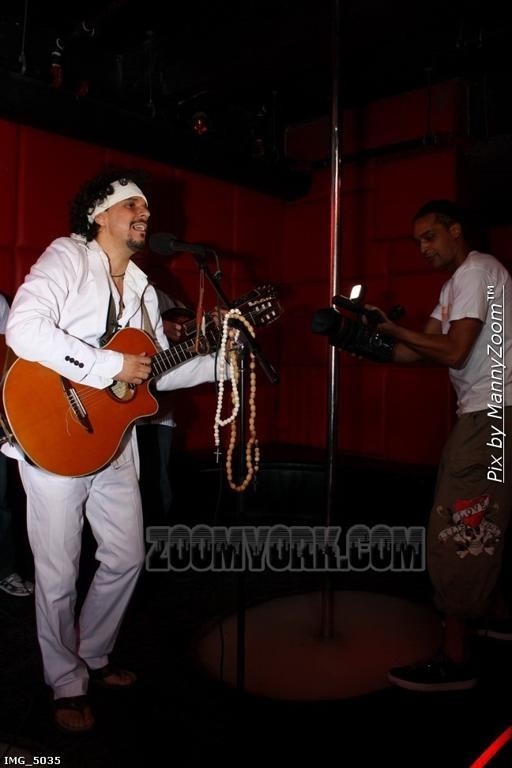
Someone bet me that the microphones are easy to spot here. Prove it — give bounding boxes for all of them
[149,233,213,256]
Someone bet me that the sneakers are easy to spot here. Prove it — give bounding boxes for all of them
[1,575,35,596]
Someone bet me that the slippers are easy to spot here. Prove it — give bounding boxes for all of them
[389,654,480,691]
[54,698,93,732]
[92,663,137,688]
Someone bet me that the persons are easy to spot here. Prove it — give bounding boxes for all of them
[338,199,512,691]
[1,167,240,732]
[0,294,34,597]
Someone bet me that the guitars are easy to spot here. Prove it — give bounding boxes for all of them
[162,284,276,349]
[1,292,284,477]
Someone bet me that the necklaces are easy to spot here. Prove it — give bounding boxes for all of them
[111,273,125,277]
